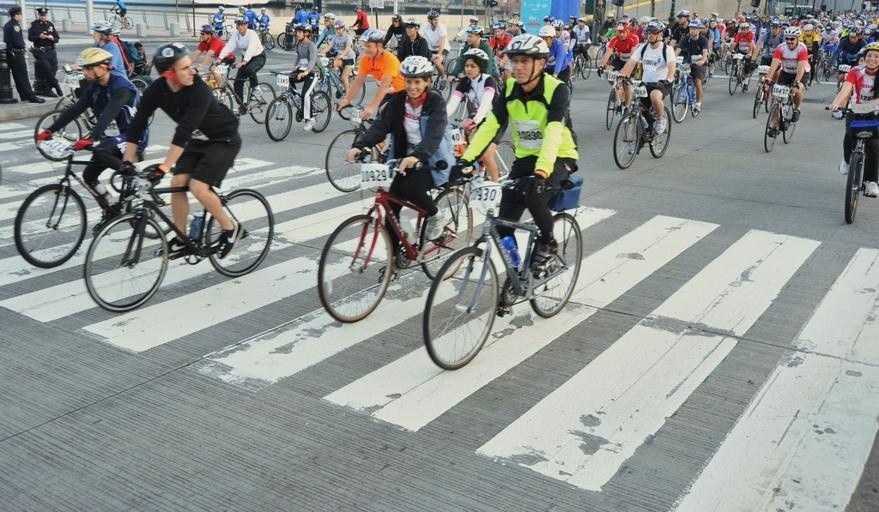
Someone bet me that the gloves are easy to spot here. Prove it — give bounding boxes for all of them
[120,159,166,182]
[596,65,606,78]
[36,129,54,142]
[515,172,546,199]
[463,117,477,133]
[72,138,94,152]
[450,160,475,187]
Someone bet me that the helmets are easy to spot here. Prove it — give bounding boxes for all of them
[154,43,193,79]
[88,19,125,38]
[499,33,554,63]
[465,13,586,40]
[356,27,385,43]
[862,41,879,58]
[616,7,878,38]
[396,54,437,79]
[291,4,361,34]
[199,4,268,34]
[76,47,114,68]
[390,7,439,30]
[459,46,490,60]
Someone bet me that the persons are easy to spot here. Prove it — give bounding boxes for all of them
[32,45,152,241]
[27,6,65,100]
[2,4,46,105]
[117,43,250,262]
[447,33,580,320]
[345,54,458,286]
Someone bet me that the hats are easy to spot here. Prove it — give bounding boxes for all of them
[35,7,51,15]
[8,6,21,14]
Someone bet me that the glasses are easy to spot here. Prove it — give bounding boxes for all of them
[784,37,796,42]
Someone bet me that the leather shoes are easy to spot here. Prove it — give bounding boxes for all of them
[23,78,64,103]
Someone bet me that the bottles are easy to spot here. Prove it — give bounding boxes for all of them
[92,181,114,202]
[191,216,202,240]
[502,237,522,266]
[400,216,417,245]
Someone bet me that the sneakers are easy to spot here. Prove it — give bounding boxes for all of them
[153,237,191,257]
[839,158,852,177]
[531,237,559,272]
[654,116,666,135]
[791,106,801,121]
[376,260,401,285]
[93,211,123,233]
[226,88,319,133]
[425,204,452,242]
[215,219,248,260]
[860,179,879,199]
[692,104,701,113]
